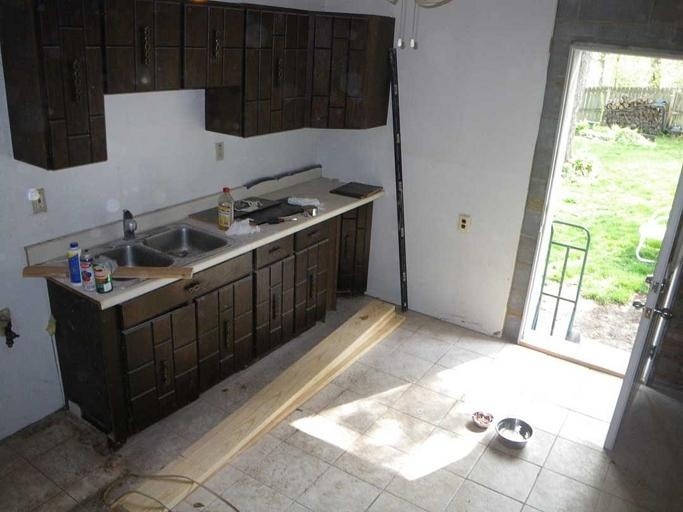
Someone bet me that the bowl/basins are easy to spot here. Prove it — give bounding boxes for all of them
[473,410,494,428]
[495,417,534,448]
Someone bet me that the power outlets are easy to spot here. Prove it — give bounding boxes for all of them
[215,141,225,160]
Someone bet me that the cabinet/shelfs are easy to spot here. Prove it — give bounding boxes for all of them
[294,220,325,338]
[325,200,375,308]
[0,1,106,172]
[46,250,257,444]
[250,233,295,356]
[308,11,396,131]
[181,1,247,92]
[101,0,182,95]
[203,4,309,138]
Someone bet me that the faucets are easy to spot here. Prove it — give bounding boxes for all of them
[121,209,136,240]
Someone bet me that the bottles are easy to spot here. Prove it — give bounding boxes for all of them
[66,240,81,290]
[217,187,235,231]
[80,248,96,292]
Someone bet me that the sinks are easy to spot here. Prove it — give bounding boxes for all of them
[141,225,230,259]
[82,244,175,283]
